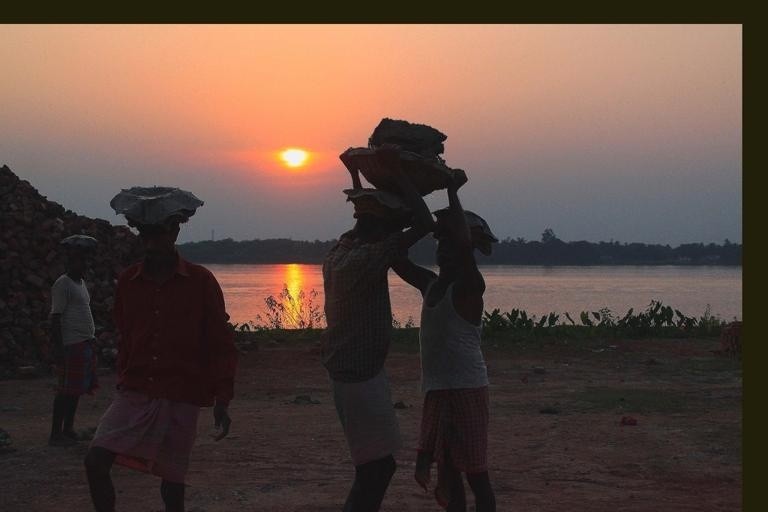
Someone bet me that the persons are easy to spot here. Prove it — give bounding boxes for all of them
[387,167,501,512]
[45,235,96,448]
[81,219,237,510]
[319,141,437,510]
